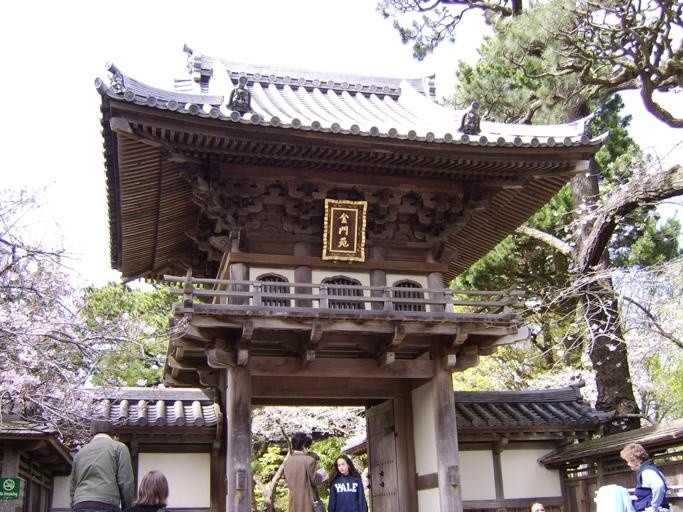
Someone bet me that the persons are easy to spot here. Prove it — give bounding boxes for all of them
[328,454,367,512]
[126,470,170,512]
[530,501,545,512]
[619,443,670,512]
[283,432,326,512]
[67,415,135,511]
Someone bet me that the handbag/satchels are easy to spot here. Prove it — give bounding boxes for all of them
[312,499,325,511]
